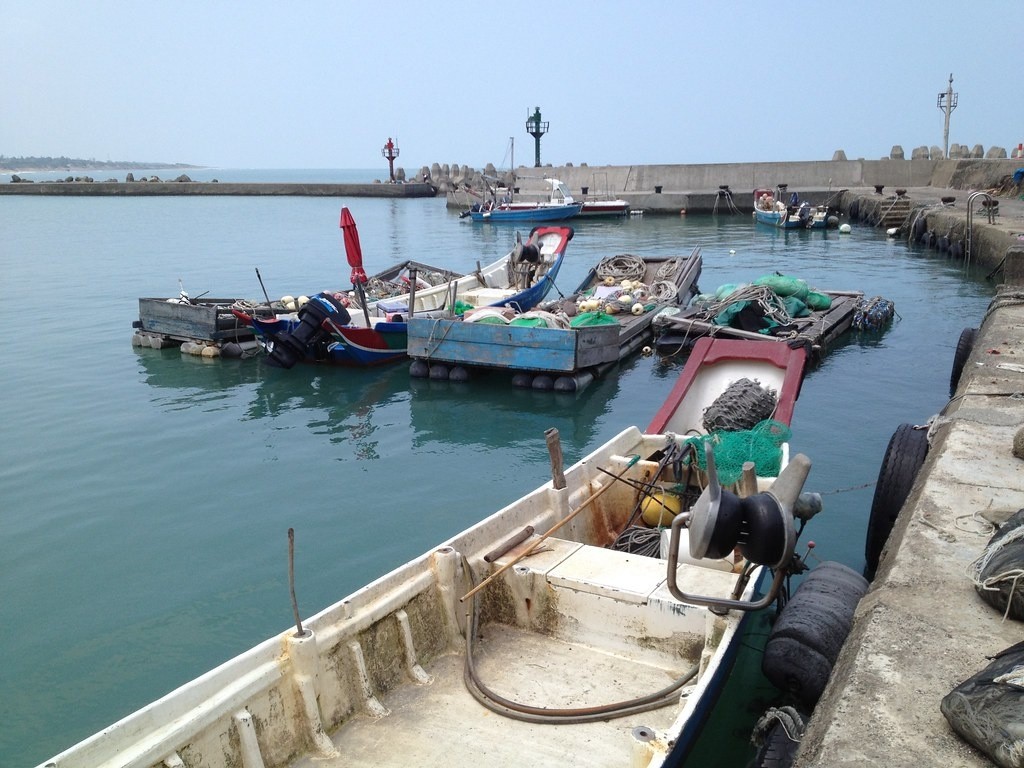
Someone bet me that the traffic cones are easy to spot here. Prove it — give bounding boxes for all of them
[1014,144,1024,159]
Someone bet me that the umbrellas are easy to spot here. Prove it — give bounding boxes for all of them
[338,205,370,287]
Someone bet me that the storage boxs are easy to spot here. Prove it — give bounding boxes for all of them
[376,301,409,317]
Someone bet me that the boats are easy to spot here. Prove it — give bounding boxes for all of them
[451,137,631,217]
[463,174,581,222]
[650,272,894,354]
[232,227,575,367]
[132,260,465,357]
[754,188,833,229]
[407,245,702,393]
[36,335,824,768]
